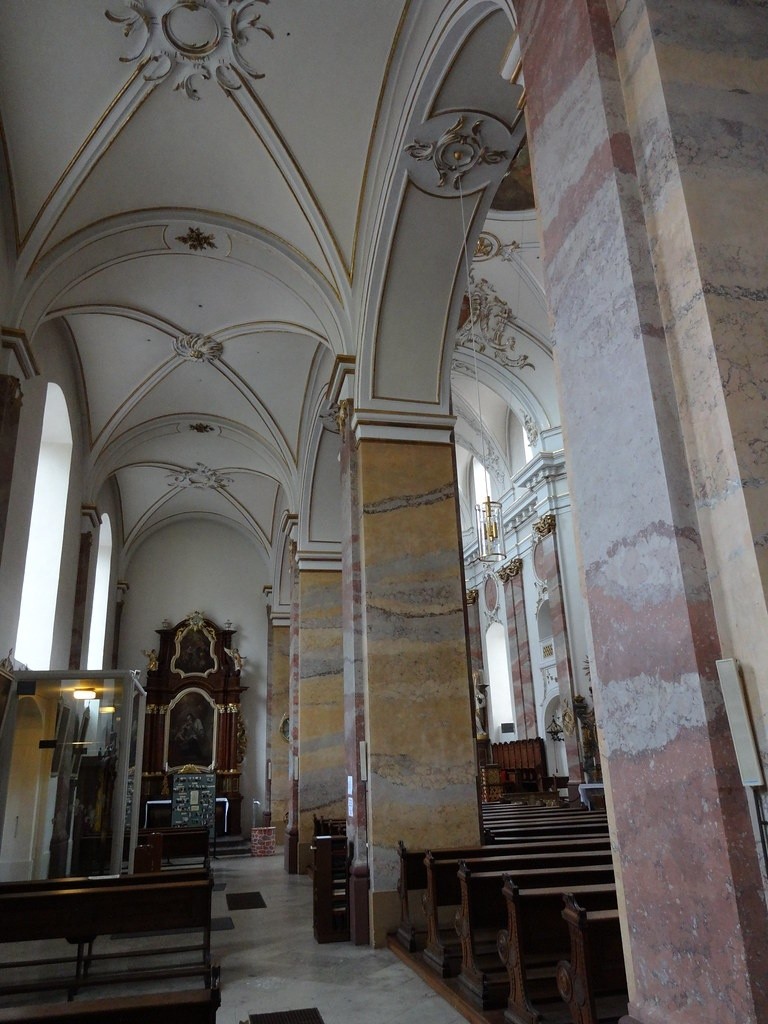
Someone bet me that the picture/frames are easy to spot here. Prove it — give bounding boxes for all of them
[51,704,72,776]
[69,706,90,778]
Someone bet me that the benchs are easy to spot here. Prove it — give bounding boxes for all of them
[0,821,223,1024]
[310,801,628,1024]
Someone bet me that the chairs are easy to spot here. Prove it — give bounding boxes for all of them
[491,737,548,793]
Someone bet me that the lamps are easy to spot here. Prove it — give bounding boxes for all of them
[451,151,509,564]
